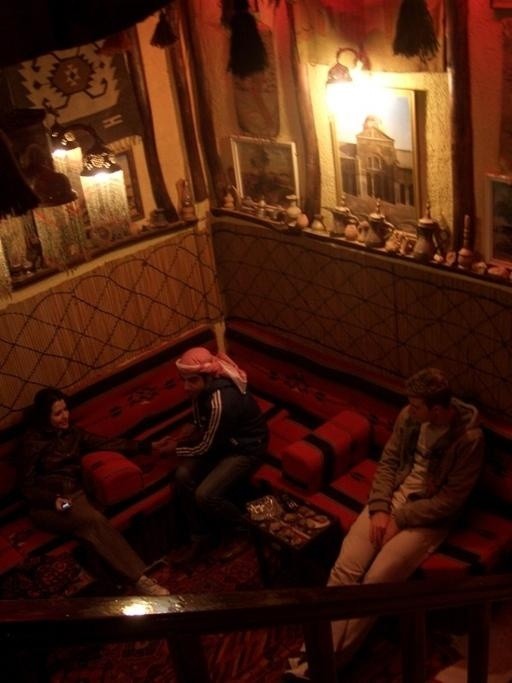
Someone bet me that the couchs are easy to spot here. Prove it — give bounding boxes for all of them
[1,390,287,577]
[236,403,512,593]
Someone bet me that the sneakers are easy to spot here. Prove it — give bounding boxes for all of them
[282,660,310,681]
[287,656,306,668]
[136,575,170,596]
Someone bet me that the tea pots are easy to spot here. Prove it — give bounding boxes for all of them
[400,199,451,262]
[357,197,396,247]
[318,193,359,241]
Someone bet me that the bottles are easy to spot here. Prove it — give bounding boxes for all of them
[256,193,268,218]
[179,179,197,218]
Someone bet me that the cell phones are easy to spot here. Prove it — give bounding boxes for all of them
[60,502,71,510]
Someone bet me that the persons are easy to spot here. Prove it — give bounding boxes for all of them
[15,388,171,597]
[159,347,270,564]
[272,368,481,680]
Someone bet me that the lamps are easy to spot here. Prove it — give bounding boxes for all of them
[42,108,125,188]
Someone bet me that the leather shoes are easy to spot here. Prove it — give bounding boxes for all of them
[189,540,202,560]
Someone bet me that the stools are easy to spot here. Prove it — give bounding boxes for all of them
[242,496,330,592]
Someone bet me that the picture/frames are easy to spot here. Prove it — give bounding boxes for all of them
[229,133,300,217]
[327,89,418,236]
